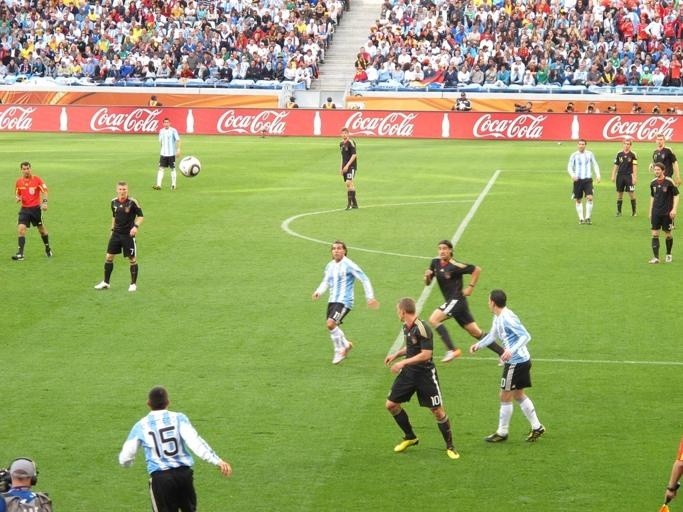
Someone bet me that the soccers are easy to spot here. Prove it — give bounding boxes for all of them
[179,155,201,177]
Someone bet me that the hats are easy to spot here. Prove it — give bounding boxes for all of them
[10,459,34,478]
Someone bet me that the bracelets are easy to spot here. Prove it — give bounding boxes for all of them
[468,284,474,287]
[665,481,680,491]
[133,223,138,229]
[41,199,47,204]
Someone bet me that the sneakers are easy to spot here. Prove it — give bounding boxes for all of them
[46,248,52,257]
[12,255,24,260]
[441,350,461,361]
[666,255,672,263]
[485,433,509,442]
[394,438,418,451]
[649,258,660,264]
[128,284,136,292]
[525,426,545,441]
[333,342,352,364]
[94,281,110,289]
[446,447,460,460]
[579,218,592,225]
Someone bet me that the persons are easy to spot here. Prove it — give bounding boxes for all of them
[469,289,546,443]
[565,103,574,112]
[647,162,680,264]
[648,133,681,228]
[567,139,601,225]
[657,436,683,512]
[0,0,350,89]
[527,102,534,112]
[117,384,232,511]
[147,96,162,107]
[611,139,638,218]
[151,118,180,191]
[338,127,358,211]
[311,240,380,364]
[287,97,298,108]
[323,97,336,108]
[586,103,617,113]
[423,240,506,366]
[353,0,683,85]
[455,92,472,111]
[9,161,53,261]
[382,297,460,459]
[0,456,52,511]
[630,103,681,113]
[93,181,144,291]
